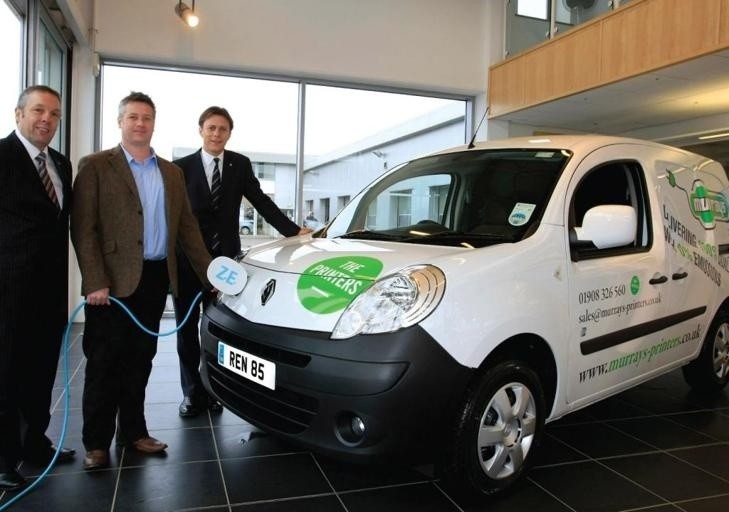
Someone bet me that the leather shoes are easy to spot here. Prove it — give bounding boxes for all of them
[132,437,167,454]
[84,450,106,468]
[0,469,26,491]
[179,396,223,417]
[49,445,75,458]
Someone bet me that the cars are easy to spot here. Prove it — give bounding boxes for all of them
[239,220,253,234]
[292,216,322,228]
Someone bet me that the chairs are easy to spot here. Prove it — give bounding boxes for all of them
[469,198,517,239]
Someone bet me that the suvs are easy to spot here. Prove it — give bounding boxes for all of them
[196,135,728,499]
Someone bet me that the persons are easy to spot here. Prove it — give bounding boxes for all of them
[0,86,76,491]
[170,106,313,417]
[70,91,217,470]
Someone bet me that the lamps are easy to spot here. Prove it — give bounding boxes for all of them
[175,0,199,27]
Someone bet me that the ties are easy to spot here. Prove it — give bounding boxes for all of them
[35,153,60,210]
[210,158,223,251]
